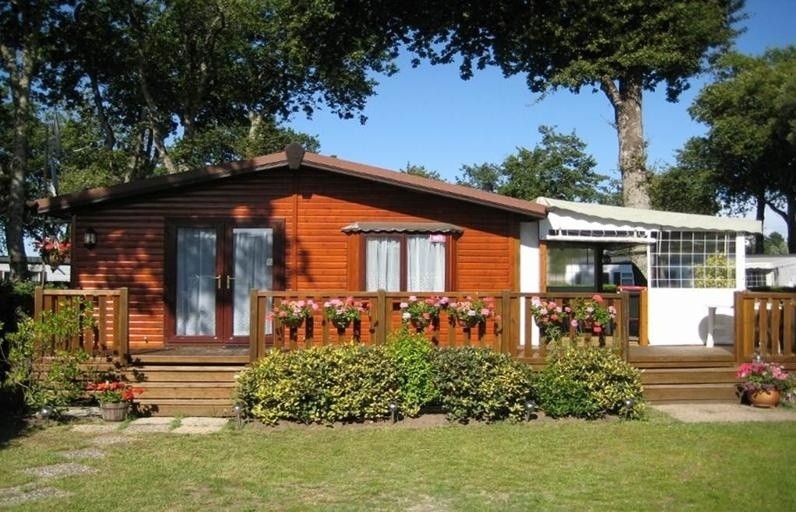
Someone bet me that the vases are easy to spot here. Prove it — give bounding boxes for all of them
[96,399,131,422]
[411,317,429,329]
[579,318,597,330]
[533,314,545,329]
[331,317,351,330]
[284,319,304,328]
[39,248,67,266]
[746,386,782,407]
[456,316,479,329]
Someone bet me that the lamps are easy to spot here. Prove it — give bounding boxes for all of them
[81,225,99,248]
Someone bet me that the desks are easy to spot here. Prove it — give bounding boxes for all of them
[696,302,785,354]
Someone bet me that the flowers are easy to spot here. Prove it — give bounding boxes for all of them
[528,294,572,330]
[399,295,448,332]
[266,295,320,328]
[448,296,500,330]
[565,295,617,333]
[733,359,796,403]
[31,229,71,258]
[321,297,371,331]
[85,381,144,403]
